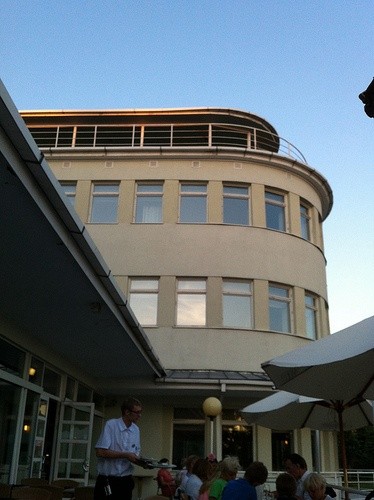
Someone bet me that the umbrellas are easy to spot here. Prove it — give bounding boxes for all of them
[261,316,374,402]
[240,390,374,500]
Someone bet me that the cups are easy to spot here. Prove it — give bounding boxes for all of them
[263,484,272,499]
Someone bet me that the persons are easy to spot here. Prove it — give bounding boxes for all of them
[95,399,158,500]
[158,453,335,499]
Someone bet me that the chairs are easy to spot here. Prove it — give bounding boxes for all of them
[153,477,174,500]
[0,476,96,500]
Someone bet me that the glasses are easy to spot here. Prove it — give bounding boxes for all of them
[129,409,143,414]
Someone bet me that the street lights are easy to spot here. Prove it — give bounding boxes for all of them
[203,397,222,454]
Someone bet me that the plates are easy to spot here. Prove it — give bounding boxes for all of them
[148,464,176,468]
